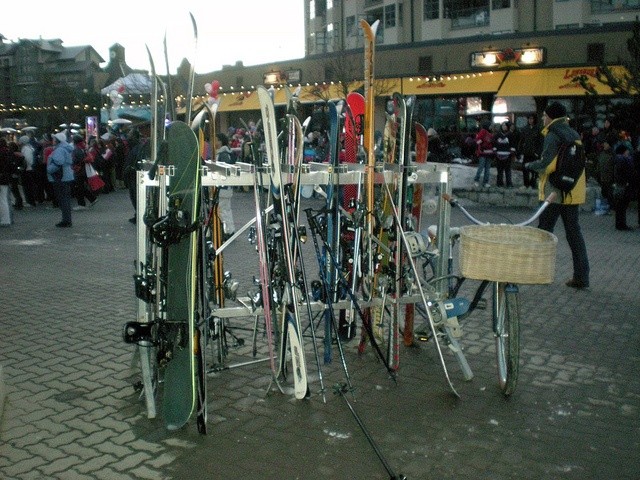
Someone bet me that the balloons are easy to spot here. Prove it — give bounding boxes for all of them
[209,89,217,98]
[212,80,220,90]
[116,85,125,94]
[208,97,216,104]
[205,83,212,94]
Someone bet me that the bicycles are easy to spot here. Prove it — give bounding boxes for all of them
[385,193,560,397]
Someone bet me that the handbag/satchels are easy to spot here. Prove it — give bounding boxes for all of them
[86,176,106,191]
[85,163,99,177]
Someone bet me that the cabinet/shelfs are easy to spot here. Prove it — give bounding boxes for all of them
[130,160,477,420]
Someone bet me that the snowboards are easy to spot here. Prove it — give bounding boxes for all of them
[157,120,199,431]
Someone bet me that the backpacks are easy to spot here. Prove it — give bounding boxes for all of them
[548,127,585,203]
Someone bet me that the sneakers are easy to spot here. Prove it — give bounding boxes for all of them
[74,206,86,211]
[485,183,490,187]
[566,278,589,288]
[89,198,98,207]
[475,181,479,186]
[55,221,71,227]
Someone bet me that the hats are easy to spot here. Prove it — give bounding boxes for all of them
[482,120,491,128]
[74,134,83,140]
[20,136,31,144]
[616,145,627,154]
[42,133,51,142]
[544,102,566,119]
[50,133,67,142]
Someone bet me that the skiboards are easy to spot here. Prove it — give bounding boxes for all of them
[283,84,302,186]
[338,91,366,342]
[359,18,381,353]
[256,85,311,400]
[163,32,175,119]
[370,111,396,344]
[185,12,197,125]
[324,100,344,364]
[202,96,225,368]
[389,91,416,370]
[276,113,312,382]
[155,74,166,140]
[403,120,430,346]
[145,43,158,161]
[239,117,284,396]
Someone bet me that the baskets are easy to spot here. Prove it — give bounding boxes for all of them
[459,223,558,285]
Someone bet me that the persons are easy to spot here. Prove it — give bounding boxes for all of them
[590,126,606,153]
[213,133,236,242]
[46,132,75,228]
[227,121,267,163]
[612,130,635,153]
[17,134,46,209]
[88,123,139,194]
[122,124,151,224]
[602,115,618,142]
[635,143,640,228]
[305,128,329,163]
[1,137,17,227]
[515,111,545,194]
[596,139,616,215]
[24,131,43,201]
[490,124,500,134]
[6,140,25,210]
[427,125,475,159]
[472,119,494,188]
[41,133,60,209]
[510,124,523,164]
[614,145,638,231]
[71,135,98,211]
[493,122,516,188]
[524,101,590,288]
